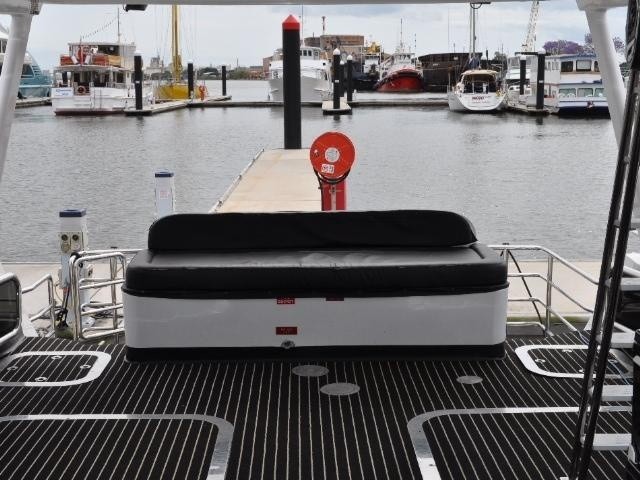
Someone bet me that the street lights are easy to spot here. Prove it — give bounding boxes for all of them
[222,63,227,96]
[133,50,144,110]
[332,48,341,109]
[186,58,194,98]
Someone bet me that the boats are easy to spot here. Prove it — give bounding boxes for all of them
[0,23,52,108]
[265,5,333,103]
[340,1,627,116]
[50,7,154,113]
[154,5,205,101]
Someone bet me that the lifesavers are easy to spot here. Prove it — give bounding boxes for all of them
[76,86,85,95]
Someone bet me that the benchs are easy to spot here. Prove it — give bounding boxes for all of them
[122,212,508,357]
